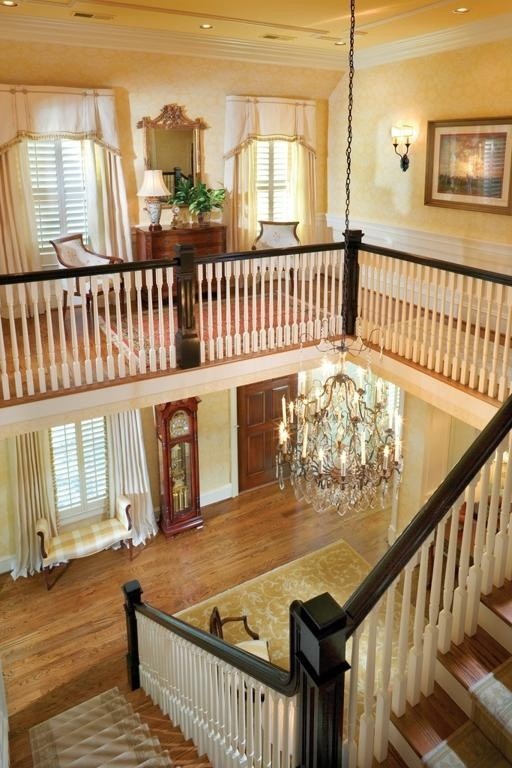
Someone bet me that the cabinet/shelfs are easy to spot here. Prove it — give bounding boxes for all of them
[134,222,226,309]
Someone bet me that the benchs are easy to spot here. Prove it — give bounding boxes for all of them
[33,494,135,591]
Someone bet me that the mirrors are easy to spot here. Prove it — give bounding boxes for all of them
[136,102,211,209]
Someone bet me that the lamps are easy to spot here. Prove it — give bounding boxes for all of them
[273,1,405,515]
[390,125,414,173]
[136,169,172,231]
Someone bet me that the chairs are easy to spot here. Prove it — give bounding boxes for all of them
[48,234,127,336]
[210,607,272,702]
[252,221,302,296]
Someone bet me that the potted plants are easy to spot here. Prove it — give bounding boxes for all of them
[188,184,226,228]
[166,175,194,206]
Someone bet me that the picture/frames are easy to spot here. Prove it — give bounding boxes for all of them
[423,116,512,217]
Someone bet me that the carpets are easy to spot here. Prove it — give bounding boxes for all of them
[382,317,512,389]
[98,290,341,368]
[170,539,434,761]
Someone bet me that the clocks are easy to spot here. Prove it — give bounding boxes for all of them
[154,396,204,541]
